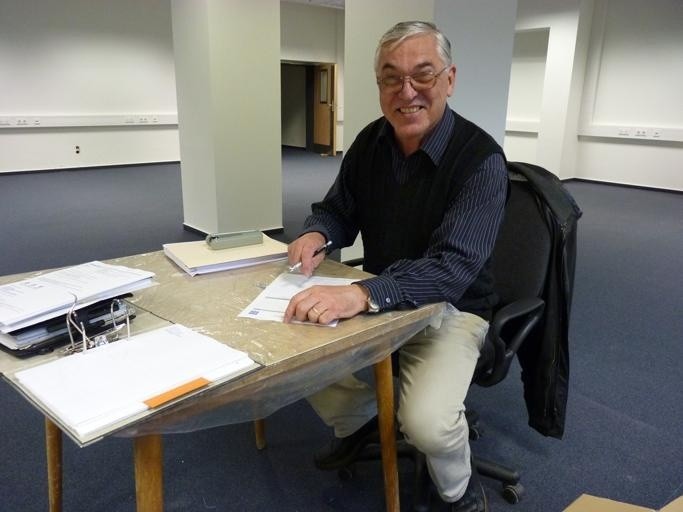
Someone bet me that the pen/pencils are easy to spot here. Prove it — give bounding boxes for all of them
[289,240,333,273]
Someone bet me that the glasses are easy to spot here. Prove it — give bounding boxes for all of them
[375,65,449,96]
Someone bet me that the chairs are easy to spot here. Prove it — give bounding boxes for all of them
[338,167,552,510]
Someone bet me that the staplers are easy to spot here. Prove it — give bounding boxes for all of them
[205,230,263,250]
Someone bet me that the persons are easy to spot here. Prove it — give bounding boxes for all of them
[282,16,509,511]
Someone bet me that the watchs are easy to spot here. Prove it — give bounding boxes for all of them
[365,295,379,315]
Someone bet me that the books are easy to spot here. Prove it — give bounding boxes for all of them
[0,260,161,359]
[161,231,289,278]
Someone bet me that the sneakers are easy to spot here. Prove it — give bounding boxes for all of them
[311,414,379,470]
[422,452,487,512]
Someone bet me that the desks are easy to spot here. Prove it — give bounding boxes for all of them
[1,250,446,511]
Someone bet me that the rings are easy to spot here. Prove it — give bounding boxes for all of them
[312,306,320,316]
